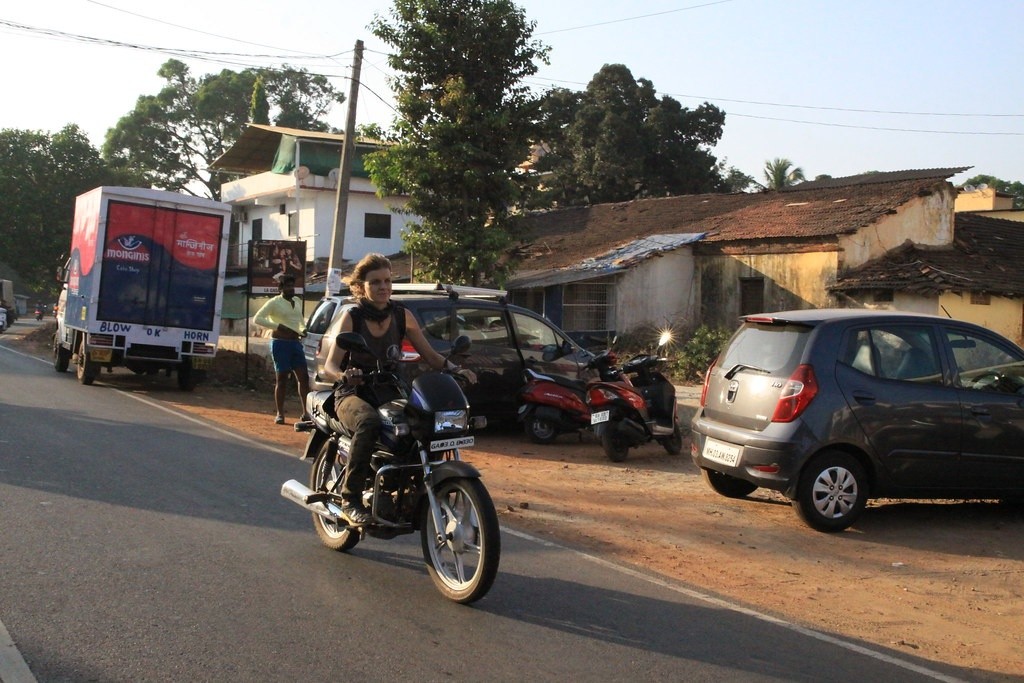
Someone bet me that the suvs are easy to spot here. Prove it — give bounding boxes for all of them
[688,306,1024,537]
[303,282,620,438]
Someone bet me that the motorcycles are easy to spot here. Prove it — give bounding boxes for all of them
[279,332,501,605]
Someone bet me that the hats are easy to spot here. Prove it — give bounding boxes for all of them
[278,275,297,287]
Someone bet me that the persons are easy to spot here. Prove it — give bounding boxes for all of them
[253,275,312,424]
[323,253,478,527]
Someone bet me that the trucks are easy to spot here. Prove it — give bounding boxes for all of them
[51,186,233,391]
[0,279,18,326]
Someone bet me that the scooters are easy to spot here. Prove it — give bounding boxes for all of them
[586,330,683,464]
[34,304,47,322]
[0,307,9,333]
[515,335,633,446]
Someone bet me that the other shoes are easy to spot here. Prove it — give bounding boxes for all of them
[300,414,311,421]
[275,416,284,423]
[340,500,372,523]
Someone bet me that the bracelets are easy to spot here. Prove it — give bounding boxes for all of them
[450,366,464,374]
